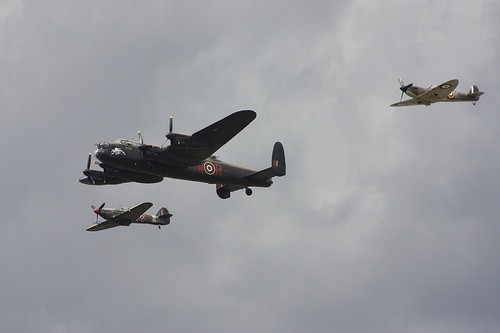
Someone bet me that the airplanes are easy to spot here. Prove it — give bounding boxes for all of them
[85,201,172,233]
[77,109,287,200]
[389,77,484,108]
